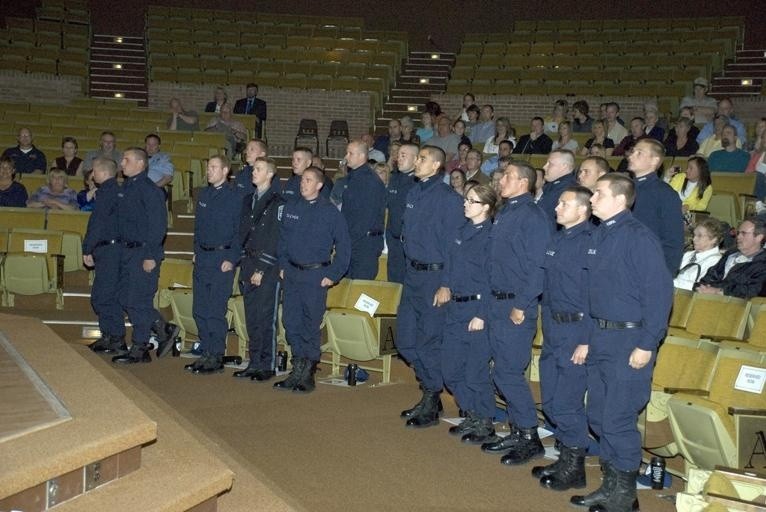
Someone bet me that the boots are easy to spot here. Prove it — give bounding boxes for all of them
[401,390,442,426]
[182,347,319,392]
[89,317,182,364]
[450,413,639,512]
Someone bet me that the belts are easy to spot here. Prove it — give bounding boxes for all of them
[96,237,147,249]
[365,230,403,242]
[198,243,231,251]
[411,260,443,271]
[452,293,481,301]
[492,290,516,299]
[288,259,329,270]
[550,311,584,322]
[598,318,642,329]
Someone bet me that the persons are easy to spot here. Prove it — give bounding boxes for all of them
[390,142,466,429]
[569,171,675,512]
[437,181,498,444]
[0,75,765,397]
[479,160,552,465]
[529,185,599,490]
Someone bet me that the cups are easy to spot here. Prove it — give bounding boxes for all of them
[278,351,287,371]
[651,457,666,489]
[173,336,181,357]
[348,363,357,386]
